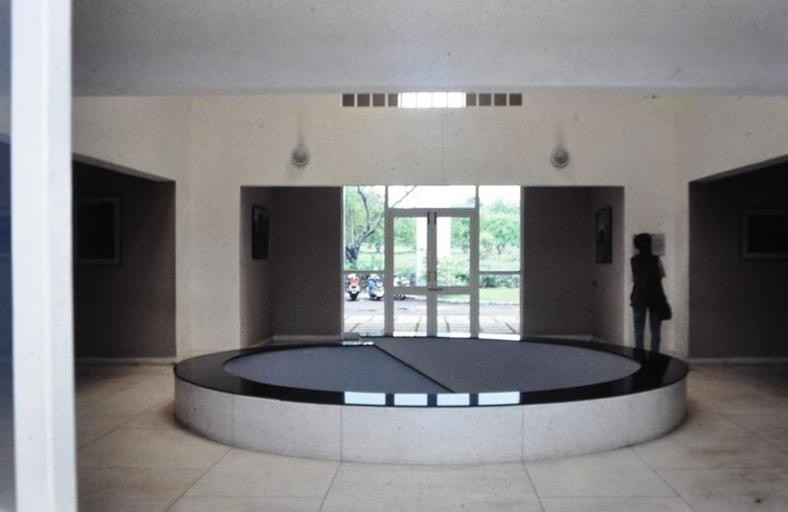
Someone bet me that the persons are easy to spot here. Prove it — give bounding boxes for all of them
[628,231,674,354]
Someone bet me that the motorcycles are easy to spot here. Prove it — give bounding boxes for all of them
[344,271,411,302]
